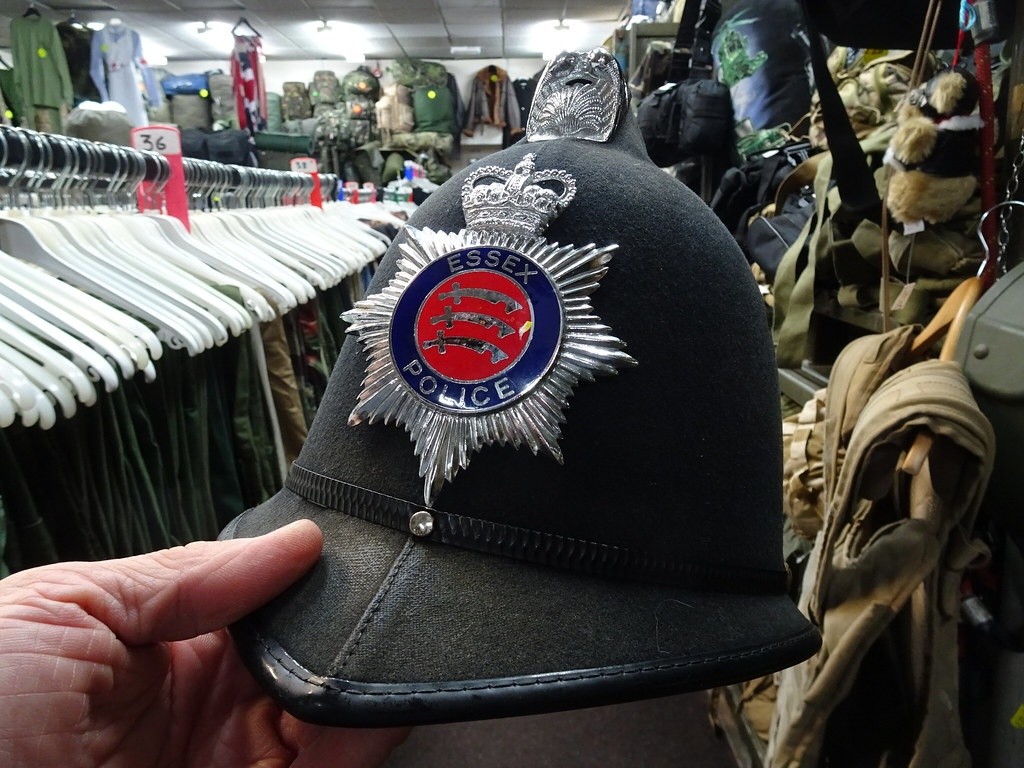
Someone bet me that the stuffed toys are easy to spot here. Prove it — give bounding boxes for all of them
[883,67,987,223]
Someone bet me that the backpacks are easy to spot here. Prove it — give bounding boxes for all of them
[150,55,453,186]
[636,1,938,313]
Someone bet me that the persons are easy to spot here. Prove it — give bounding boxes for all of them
[0,517,420,768]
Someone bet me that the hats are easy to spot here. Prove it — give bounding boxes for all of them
[220,50,823,725]
